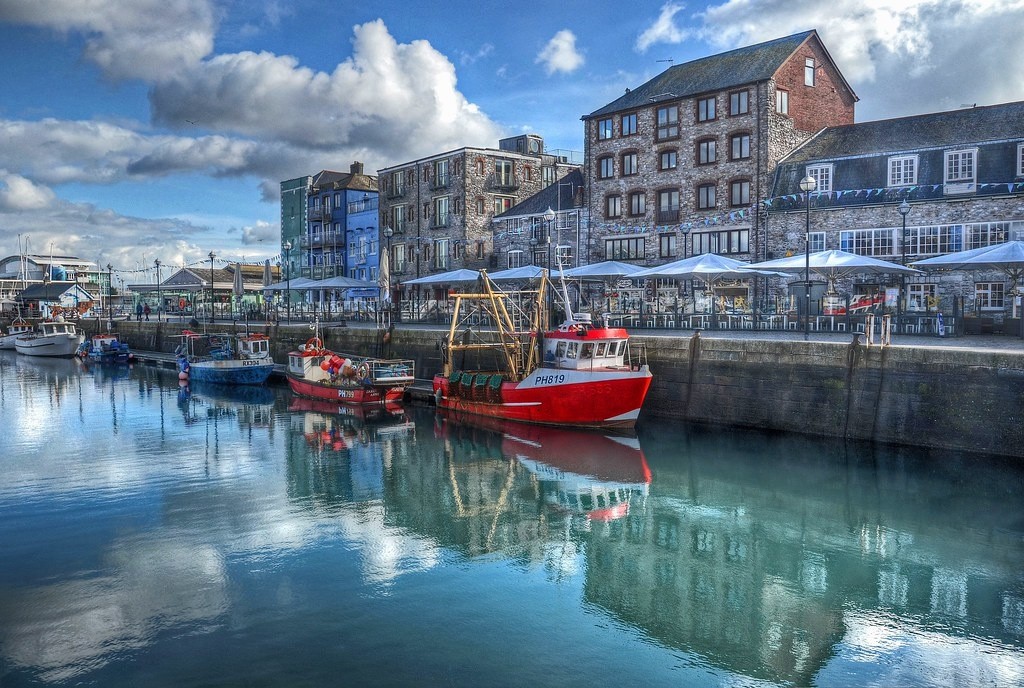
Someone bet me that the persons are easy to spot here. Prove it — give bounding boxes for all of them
[144,303,151,321]
[136,302,143,321]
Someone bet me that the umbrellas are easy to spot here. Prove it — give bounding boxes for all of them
[232,263,244,323]
[400,269,483,312]
[483,264,562,309]
[263,259,275,322]
[290,275,378,324]
[562,260,650,292]
[379,247,391,328]
[622,252,793,315]
[262,276,322,321]
[737,249,927,316]
[905,240,1024,318]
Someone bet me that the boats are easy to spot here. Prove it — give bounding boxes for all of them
[429,410,656,538]
[85,361,134,432]
[176,377,278,477]
[164,330,278,386]
[282,386,418,452]
[73,333,133,364]
[14,348,85,424]
[14,318,85,358]
[432,265,654,426]
[282,312,416,416]
[0,303,33,351]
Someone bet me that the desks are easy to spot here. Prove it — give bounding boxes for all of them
[770,315,788,330]
[690,315,711,329]
[652,314,673,328]
[728,315,750,329]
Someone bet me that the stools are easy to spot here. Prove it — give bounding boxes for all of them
[612,320,954,334]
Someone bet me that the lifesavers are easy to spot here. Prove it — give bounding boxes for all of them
[305,337,323,355]
[355,362,370,381]
[180,298,185,309]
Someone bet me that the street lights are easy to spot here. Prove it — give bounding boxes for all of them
[412,246,421,306]
[107,263,114,320]
[528,236,539,266]
[209,251,217,322]
[275,262,281,313]
[154,258,162,321]
[543,206,555,330]
[284,240,294,324]
[679,225,691,315]
[798,171,820,339]
[382,225,393,325]
[895,197,912,299]
